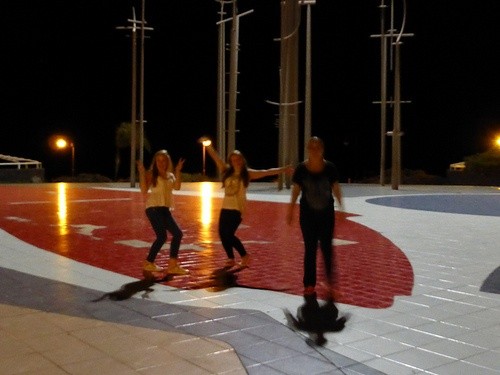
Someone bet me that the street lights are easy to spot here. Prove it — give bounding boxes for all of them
[202,140,211,175]
[56,140,75,178]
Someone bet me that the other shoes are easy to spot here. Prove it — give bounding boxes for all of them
[143,260,164,272]
[167,265,190,274]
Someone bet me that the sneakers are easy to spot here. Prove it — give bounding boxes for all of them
[238,257,253,267]
[222,259,236,268]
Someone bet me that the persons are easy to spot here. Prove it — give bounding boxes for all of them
[138,150,190,275]
[201,138,292,267]
[285,137,343,297]
